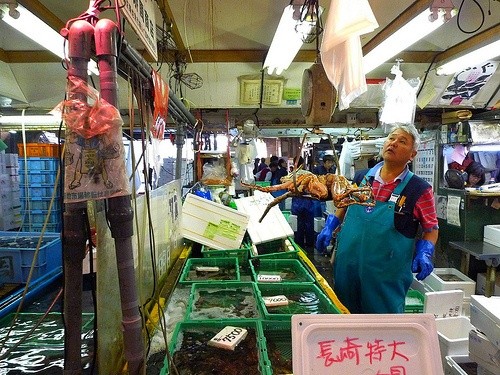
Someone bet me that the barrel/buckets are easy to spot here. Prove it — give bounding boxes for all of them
[207,185,226,197]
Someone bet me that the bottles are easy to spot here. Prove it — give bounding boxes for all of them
[450,130,457,143]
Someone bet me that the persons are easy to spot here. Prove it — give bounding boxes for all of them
[465,162,486,185]
[316,124,439,314]
[253,155,336,253]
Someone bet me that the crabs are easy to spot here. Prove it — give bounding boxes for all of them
[240,164,376,222]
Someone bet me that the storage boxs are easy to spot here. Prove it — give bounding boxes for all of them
[0,142,94,356]
[157,181,500,375]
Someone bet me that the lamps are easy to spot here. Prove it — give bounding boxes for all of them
[362,0,500,76]
[262,0,325,75]
[0,0,100,76]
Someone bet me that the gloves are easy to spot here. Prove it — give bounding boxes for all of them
[316,215,340,253]
[412,239,434,281]
